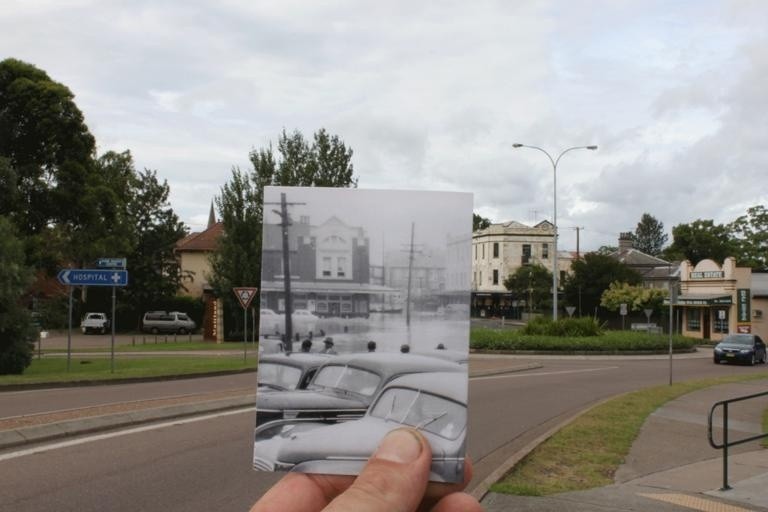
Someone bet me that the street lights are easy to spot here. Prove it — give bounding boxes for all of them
[511,141,599,322]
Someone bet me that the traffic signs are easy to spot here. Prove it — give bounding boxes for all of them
[57,268,128,286]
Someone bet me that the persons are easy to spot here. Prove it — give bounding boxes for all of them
[307,332,314,339]
[295,332,300,341]
[320,329,326,338]
[299,340,312,352]
[435,342,447,349]
[249,429,485,512]
[319,337,339,355]
[399,345,410,352]
[366,341,376,352]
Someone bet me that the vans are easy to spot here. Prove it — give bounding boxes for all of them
[142,310,198,335]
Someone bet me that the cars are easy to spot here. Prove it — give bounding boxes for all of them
[254,349,469,483]
[713,333,766,366]
[80,312,110,334]
